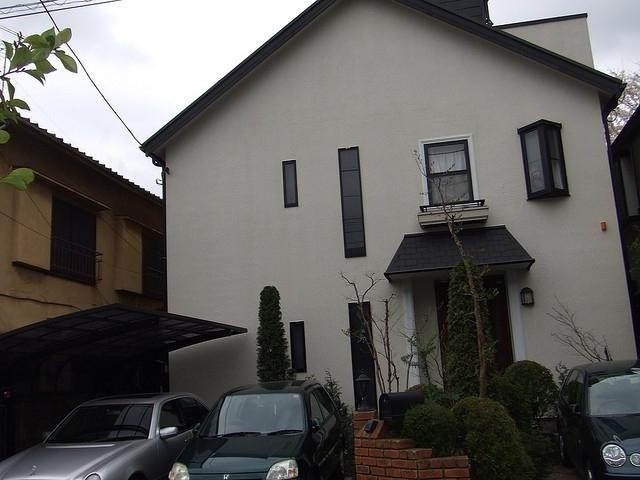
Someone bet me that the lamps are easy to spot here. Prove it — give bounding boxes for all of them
[519,287,535,307]
[354,369,374,413]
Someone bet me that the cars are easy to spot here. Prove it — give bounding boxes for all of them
[557,360,640,479]
[171,379,346,480]
[1,390,210,480]
